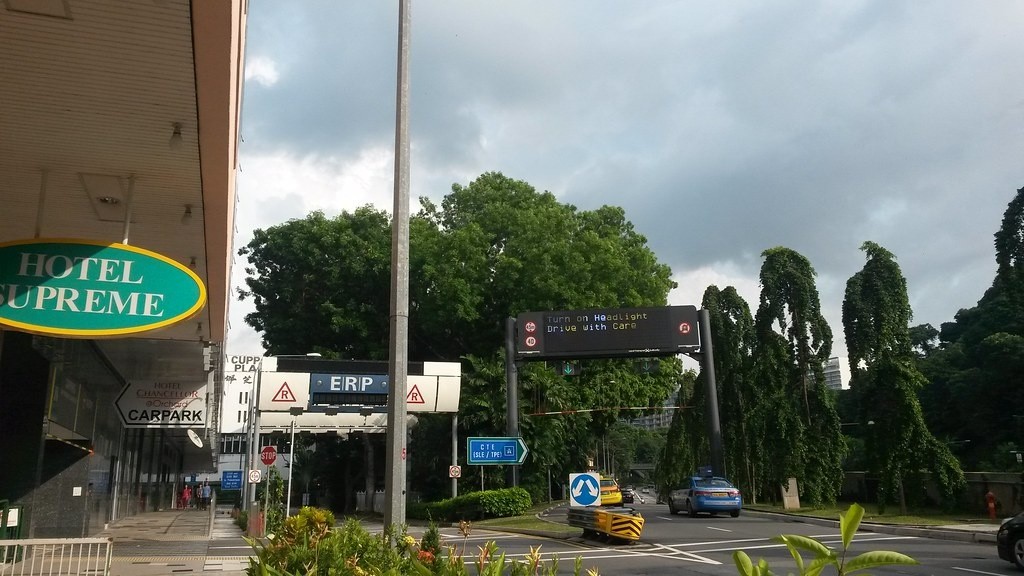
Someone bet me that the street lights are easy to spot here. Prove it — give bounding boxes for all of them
[602,419,627,475]
[607,438,627,477]
[245,351,323,508]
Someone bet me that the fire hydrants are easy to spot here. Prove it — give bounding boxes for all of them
[985,492,997,519]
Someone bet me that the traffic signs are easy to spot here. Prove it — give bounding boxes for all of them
[466,435,529,467]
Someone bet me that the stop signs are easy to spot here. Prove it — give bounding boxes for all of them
[260,446,277,466]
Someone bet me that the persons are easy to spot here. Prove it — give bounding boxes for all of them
[182,485,190,511]
[190,481,212,511]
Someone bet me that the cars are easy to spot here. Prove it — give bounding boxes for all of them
[626,485,637,491]
[621,488,635,504]
[642,486,650,494]
[668,465,742,517]
[599,474,623,506]
[995,510,1024,572]
